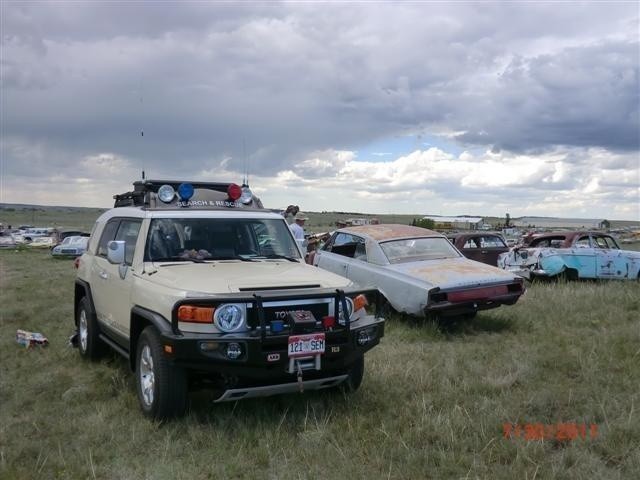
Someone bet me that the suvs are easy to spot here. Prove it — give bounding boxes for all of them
[73,179,387,423]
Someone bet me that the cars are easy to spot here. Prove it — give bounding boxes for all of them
[0,225,61,249]
[310,223,528,327]
[51,236,90,257]
[443,225,640,281]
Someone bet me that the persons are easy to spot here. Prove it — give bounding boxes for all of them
[279,204,348,266]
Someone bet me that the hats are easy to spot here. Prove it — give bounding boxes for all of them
[293,212,309,221]
[336,219,348,225]
